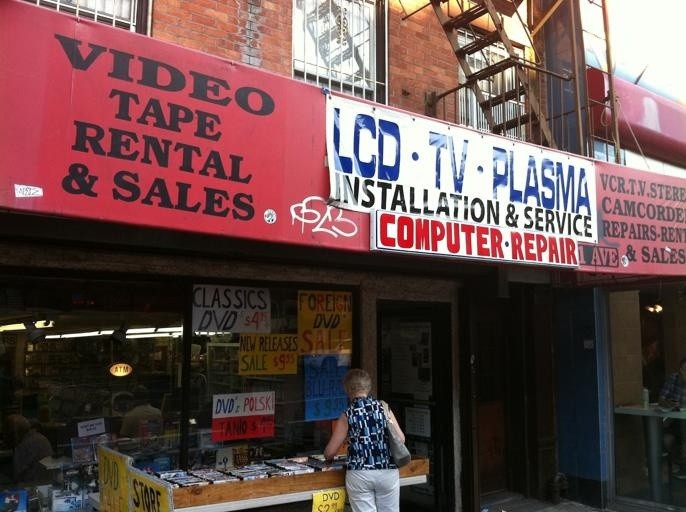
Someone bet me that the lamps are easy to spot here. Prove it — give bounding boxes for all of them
[0,320,54,332]
[125,326,230,340]
[44,330,114,339]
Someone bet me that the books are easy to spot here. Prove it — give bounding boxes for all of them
[152,426,351,490]
[1,432,151,511]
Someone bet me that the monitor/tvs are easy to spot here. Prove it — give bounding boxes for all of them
[160,393,201,411]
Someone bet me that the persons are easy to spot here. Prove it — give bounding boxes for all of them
[118,383,162,437]
[0,413,54,482]
[655,356,686,480]
[322,367,407,512]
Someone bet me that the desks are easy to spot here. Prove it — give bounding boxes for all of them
[614,403,686,503]
[88,475,430,512]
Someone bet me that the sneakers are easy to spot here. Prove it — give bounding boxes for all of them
[380,400,411,467]
